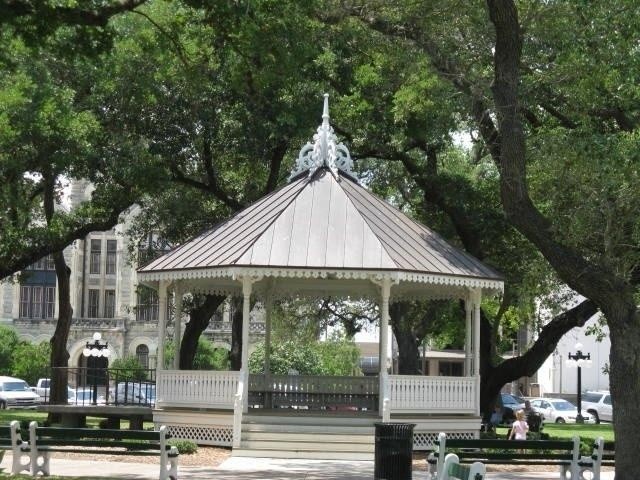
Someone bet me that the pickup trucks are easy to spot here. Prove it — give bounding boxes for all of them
[24,378,74,399]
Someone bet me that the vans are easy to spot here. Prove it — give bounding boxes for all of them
[581,391,613,424]
[0,376,41,410]
[112,382,155,406]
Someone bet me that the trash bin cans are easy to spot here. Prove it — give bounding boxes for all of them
[373,422,416,480]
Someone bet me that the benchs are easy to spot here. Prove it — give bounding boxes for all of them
[426,431,616,480]
[0,419,180,479]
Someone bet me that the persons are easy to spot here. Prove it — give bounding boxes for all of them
[507,409,530,454]
[507,400,534,440]
[480,404,504,433]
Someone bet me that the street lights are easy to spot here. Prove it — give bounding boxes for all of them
[83,332,110,405]
[566,343,592,424]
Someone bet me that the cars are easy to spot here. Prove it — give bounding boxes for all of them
[500,391,589,428]
[74,389,100,404]
